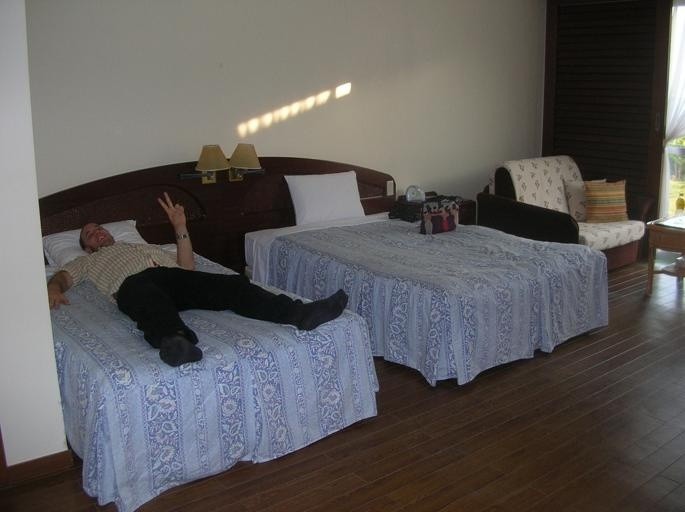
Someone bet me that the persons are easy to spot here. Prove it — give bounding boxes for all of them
[47,192,348,367]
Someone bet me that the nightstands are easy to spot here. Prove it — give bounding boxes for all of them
[399,191,475,224]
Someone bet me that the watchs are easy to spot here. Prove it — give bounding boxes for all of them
[175,231,189,240]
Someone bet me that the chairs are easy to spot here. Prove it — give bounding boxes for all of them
[475,155,654,269]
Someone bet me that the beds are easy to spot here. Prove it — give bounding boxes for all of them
[243,223,608,413]
[53,240,380,509]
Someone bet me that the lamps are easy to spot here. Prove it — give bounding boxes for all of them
[191,141,261,187]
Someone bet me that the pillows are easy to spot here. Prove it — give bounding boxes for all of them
[278,170,368,223]
[562,178,630,223]
[44,219,148,270]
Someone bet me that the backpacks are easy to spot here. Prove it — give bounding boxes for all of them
[420,199,459,234]
